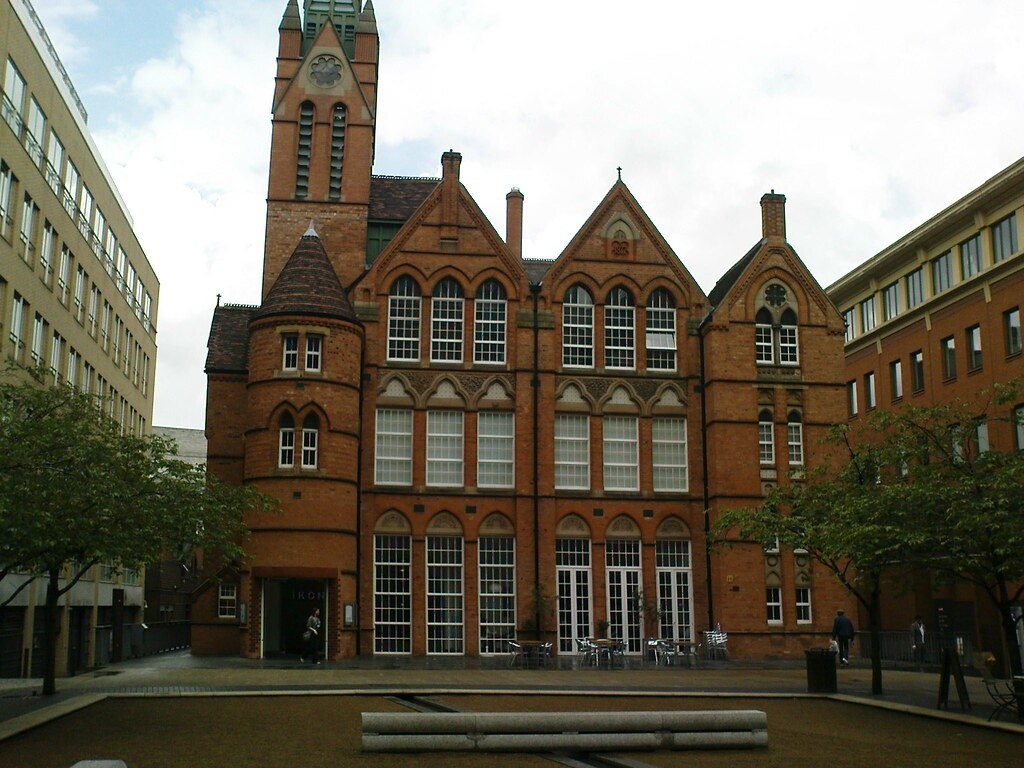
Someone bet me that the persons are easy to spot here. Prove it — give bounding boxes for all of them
[910,615,928,669]
[299,607,321,665]
[831,609,856,668]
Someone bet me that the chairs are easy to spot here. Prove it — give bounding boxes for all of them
[505,630,729,669]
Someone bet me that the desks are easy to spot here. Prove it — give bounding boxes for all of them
[670,640,701,667]
[982,678,1024,721]
[515,640,546,668]
[586,638,618,667]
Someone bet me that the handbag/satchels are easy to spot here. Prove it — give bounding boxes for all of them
[302,630,312,641]
[828,639,839,656]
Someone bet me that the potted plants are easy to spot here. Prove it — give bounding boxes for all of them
[597,619,610,660]
[635,588,665,660]
[521,585,556,658]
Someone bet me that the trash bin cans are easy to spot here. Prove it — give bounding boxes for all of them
[803,646,839,693]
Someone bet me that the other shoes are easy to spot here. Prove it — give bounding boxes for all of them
[312,662,320,665]
[298,655,305,664]
[842,657,848,664]
